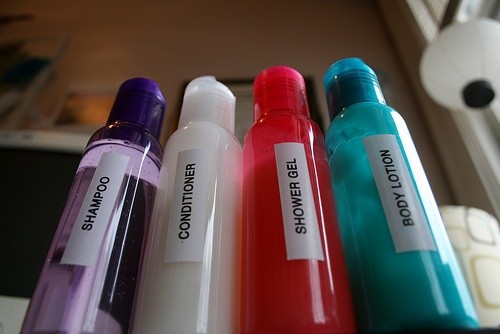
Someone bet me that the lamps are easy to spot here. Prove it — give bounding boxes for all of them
[418,17,500,114]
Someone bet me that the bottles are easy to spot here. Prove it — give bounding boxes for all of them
[124,76,242,332]
[323,55,481,329]
[17,78,167,334]
[237,65,356,334]
